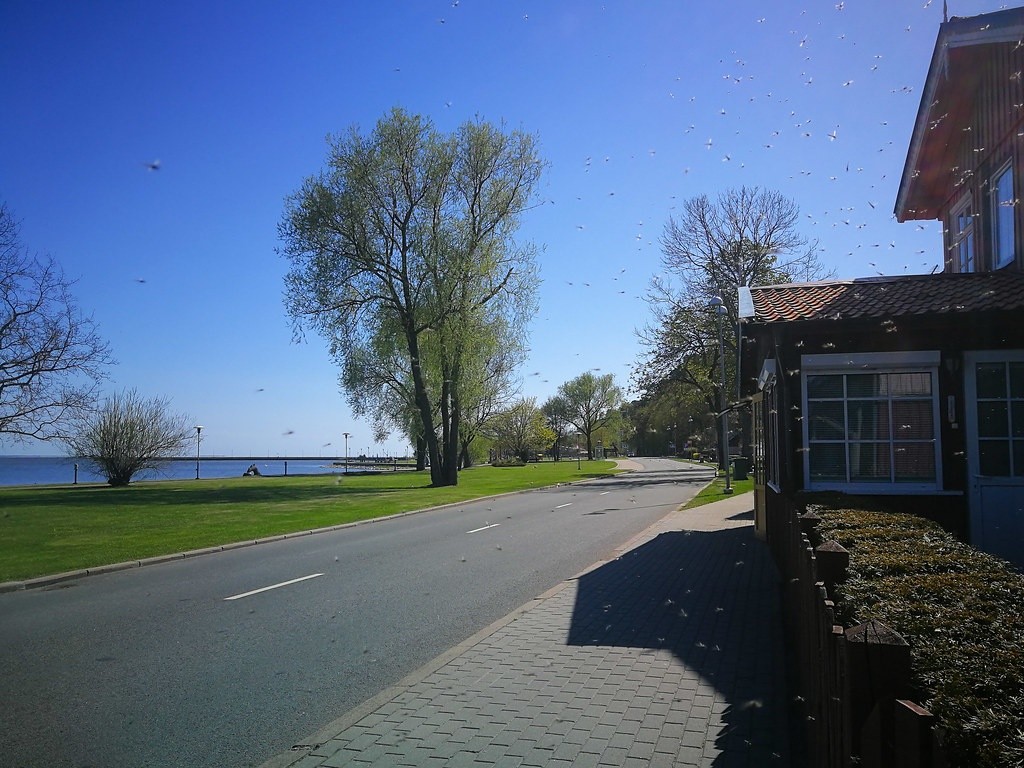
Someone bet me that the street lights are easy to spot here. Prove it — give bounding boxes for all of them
[667,425,671,458]
[342,433,350,473]
[709,296,734,494]
[574,431,583,470]
[688,415,693,464]
[194,426,204,479]
[673,422,677,459]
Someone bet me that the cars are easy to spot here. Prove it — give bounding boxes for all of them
[628,452,635,457]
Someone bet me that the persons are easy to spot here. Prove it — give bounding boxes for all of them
[246,465,254,476]
[251,463,261,476]
